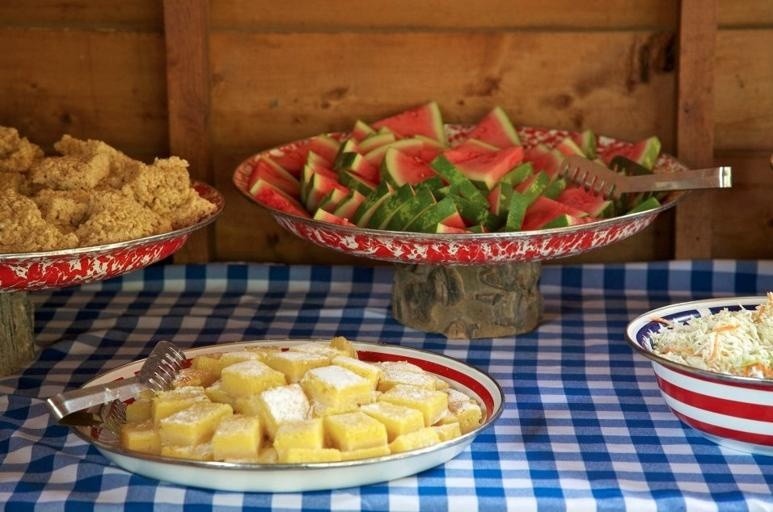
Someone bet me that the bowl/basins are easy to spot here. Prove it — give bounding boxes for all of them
[625,296,772,457]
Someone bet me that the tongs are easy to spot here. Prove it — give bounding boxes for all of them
[46,340,188,426]
[558,156,733,201]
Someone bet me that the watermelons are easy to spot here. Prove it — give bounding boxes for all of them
[249,101,662,234]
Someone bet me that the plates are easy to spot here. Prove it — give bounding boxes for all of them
[231,122,695,265]
[74,338,505,495]
[0,179,227,294]
